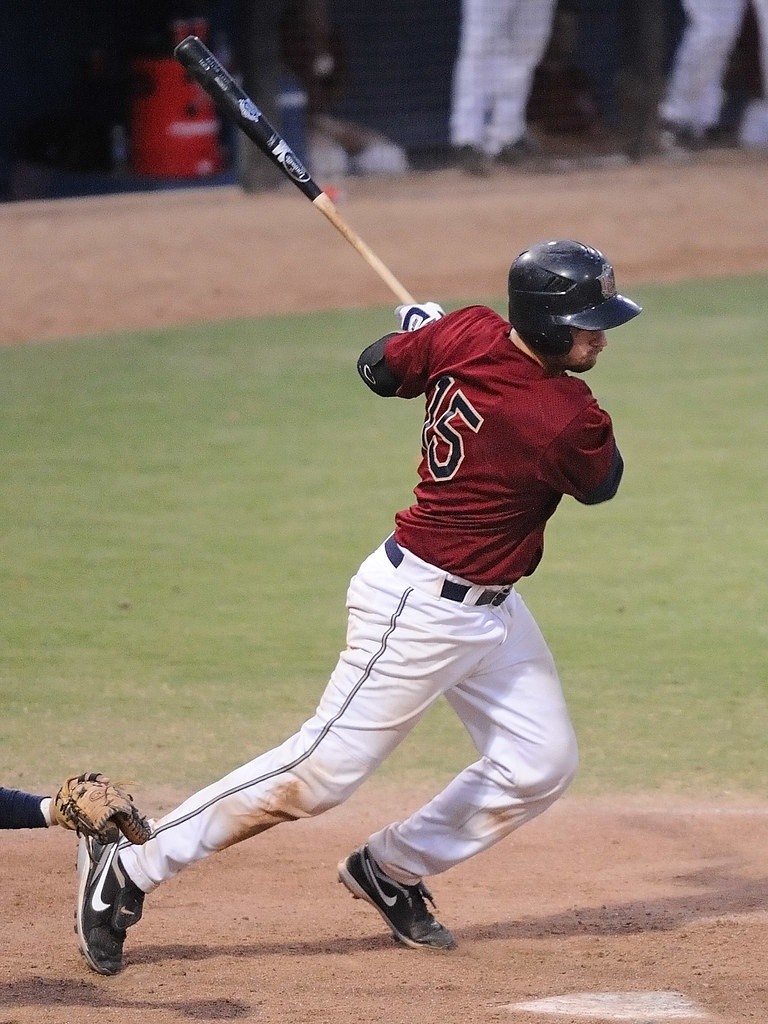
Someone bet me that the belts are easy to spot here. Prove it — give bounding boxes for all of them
[385,534,510,607]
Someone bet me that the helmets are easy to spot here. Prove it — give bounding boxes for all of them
[508,239,643,359]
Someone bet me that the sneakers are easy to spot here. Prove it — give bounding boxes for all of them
[337,843,454,949]
[74,832,146,976]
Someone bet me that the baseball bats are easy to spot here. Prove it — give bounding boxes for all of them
[172,33,421,306]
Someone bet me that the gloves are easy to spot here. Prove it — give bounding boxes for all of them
[394,301,447,332]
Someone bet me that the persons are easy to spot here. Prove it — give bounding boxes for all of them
[110,0,750,181]
[72,242,643,976]
[0,774,151,846]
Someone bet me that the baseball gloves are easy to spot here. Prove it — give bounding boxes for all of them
[52,772,153,846]
